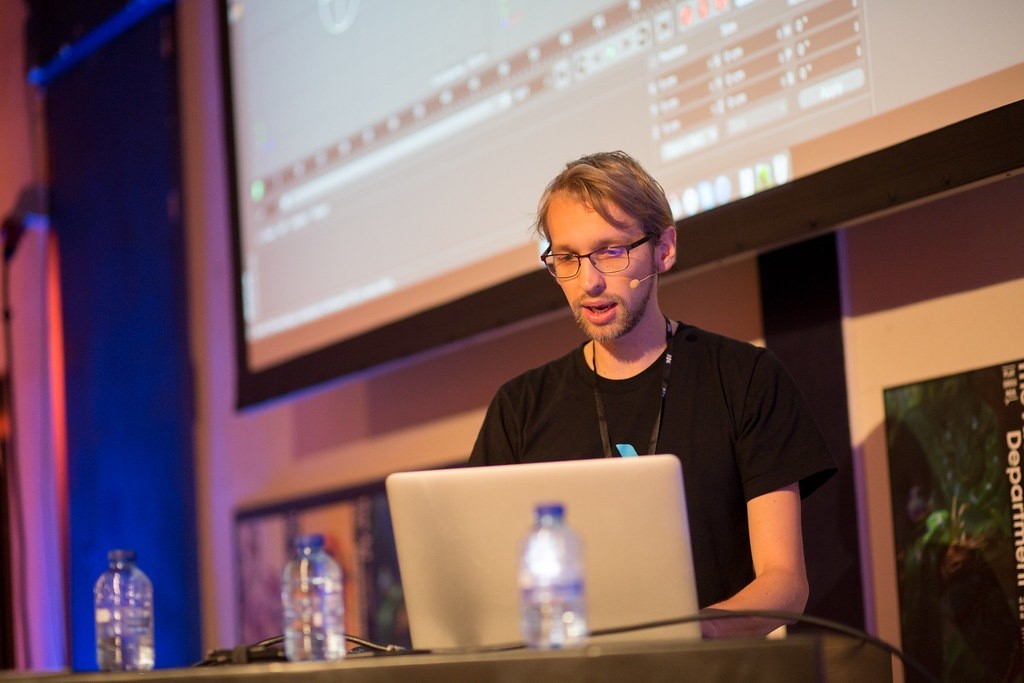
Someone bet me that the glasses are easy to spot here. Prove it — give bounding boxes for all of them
[542,237,651,279]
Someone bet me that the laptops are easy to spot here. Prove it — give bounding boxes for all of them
[387,454,702,652]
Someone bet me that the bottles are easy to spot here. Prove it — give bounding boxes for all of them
[279,533,344,660]
[94,548,155,672]
[519,502,589,651]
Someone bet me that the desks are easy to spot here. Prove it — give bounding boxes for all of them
[0,636,826,683]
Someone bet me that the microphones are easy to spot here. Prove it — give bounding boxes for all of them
[630,273,658,289]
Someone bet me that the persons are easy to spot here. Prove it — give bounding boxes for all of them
[469,151,810,640]
